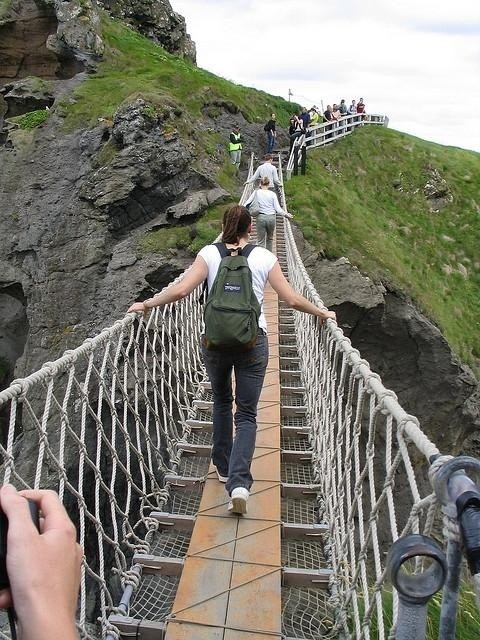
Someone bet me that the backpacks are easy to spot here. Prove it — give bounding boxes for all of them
[200,242,261,352]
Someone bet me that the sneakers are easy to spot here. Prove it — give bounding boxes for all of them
[228,487,249,514]
[214,465,229,483]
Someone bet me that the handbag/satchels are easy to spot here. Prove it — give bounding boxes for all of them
[249,199,259,217]
[301,129,305,134]
[263,123,275,131]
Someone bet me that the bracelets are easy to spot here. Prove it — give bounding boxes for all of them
[142,297,152,310]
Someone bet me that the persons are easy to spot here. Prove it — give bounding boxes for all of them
[127,204,337,516]
[263,113,277,151]
[288,97,366,153]
[0,482,84,640]
[229,125,242,168]
[241,153,293,252]
